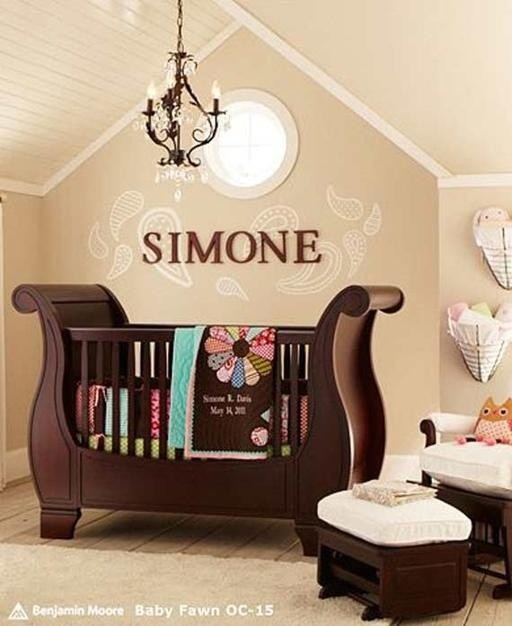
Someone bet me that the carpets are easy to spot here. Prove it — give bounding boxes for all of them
[0,543,396,626]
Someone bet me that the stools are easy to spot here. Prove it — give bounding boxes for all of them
[314,490,472,622]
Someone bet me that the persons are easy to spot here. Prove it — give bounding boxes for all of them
[455,393,512,447]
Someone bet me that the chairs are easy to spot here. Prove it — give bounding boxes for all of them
[418,411,512,602]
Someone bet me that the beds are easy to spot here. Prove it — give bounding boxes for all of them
[11,282,403,557]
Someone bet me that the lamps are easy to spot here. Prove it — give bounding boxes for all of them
[141,1,229,168]
